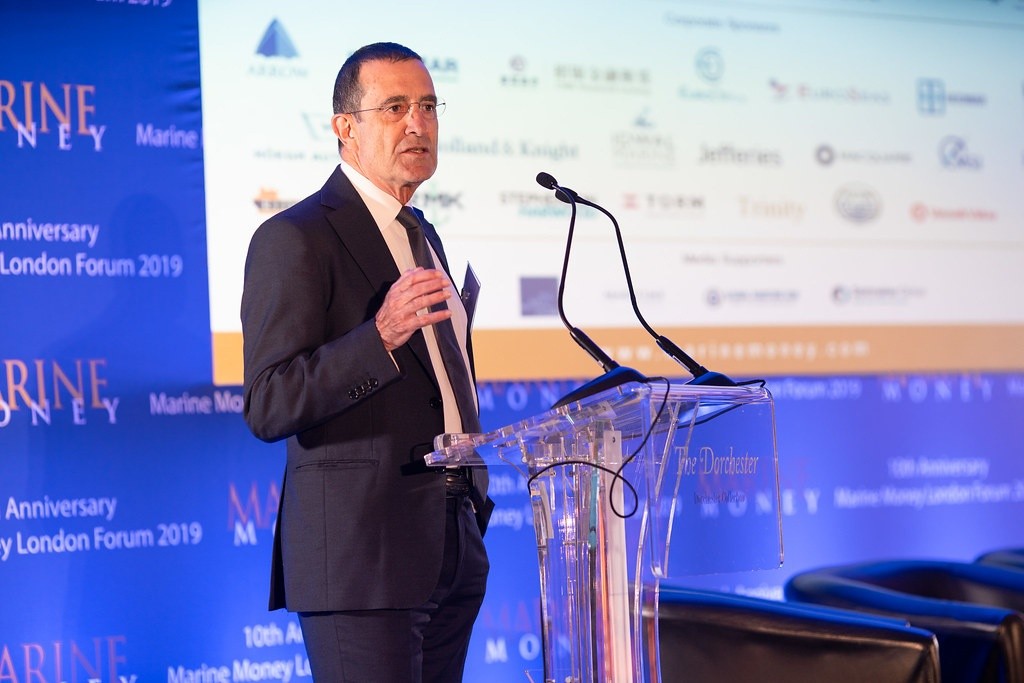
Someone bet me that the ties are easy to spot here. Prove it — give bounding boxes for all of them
[395,205,481,435]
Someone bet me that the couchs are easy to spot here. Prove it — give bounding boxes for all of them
[785,548,1024,683]
[639,586,942,683]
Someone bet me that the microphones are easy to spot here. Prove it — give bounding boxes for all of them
[557,185,742,382]
[534,173,649,411]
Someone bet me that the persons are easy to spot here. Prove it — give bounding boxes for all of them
[240,42,496,683]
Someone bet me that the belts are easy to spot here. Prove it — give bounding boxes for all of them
[444,468,473,496]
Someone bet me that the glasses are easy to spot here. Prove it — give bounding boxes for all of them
[342,98,447,120]
